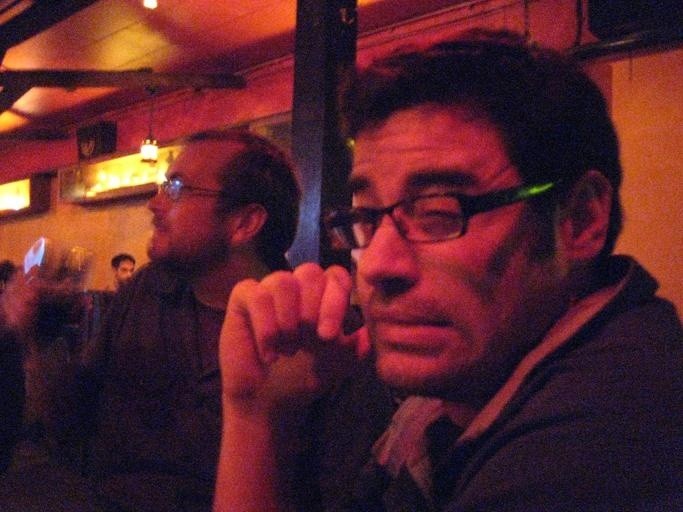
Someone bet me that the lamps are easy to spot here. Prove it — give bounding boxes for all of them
[137,85,158,164]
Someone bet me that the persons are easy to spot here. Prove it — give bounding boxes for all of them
[105,244,138,293]
[213,28,683,512]
[1,126,301,512]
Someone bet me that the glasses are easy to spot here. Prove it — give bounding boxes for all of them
[322,175,566,251]
[160,176,233,202]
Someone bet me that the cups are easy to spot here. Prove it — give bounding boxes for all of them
[27,238,98,348]
